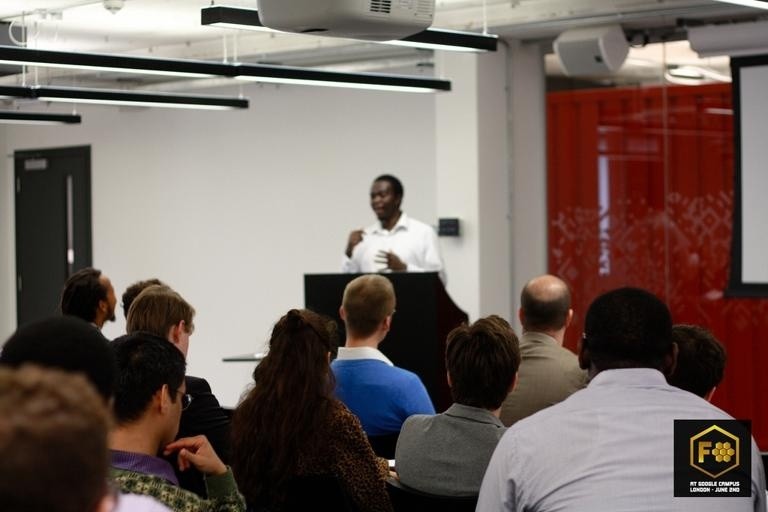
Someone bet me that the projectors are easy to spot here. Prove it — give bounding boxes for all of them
[255,0,437,45]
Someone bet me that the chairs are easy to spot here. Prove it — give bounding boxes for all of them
[382,473,493,505]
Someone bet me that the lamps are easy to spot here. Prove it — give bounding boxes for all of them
[201,6,498,54]
[1,44,237,79]
[1,109,83,126]
[3,84,34,100]
[232,63,454,96]
[37,87,251,111]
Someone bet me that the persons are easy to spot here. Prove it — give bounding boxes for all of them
[126,285,232,500]
[476,287,768,512]
[121,278,165,321]
[58,267,117,337]
[344,174,448,288]
[0,363,118,512]
[394,315,522,497]
[665,324,727,403]
[0,315,111,404]
[496,274,589,428]
[327,274,436,436]
[111,331,246,512]
[226,308,393,512]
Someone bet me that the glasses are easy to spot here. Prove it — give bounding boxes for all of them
[176,389,192,412]
